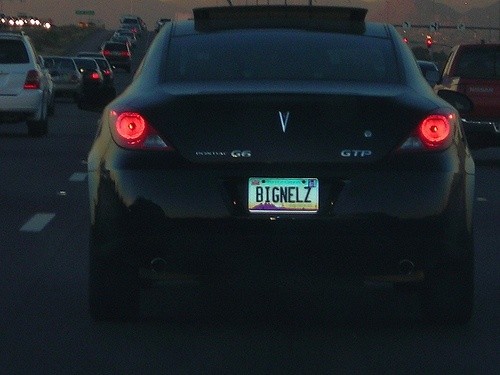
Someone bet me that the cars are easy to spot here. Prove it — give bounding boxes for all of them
[417,61,440,87]
[434,43,500,134]
[88,3,478,329]
[0,32,50,137]
[40,16,172,95]
